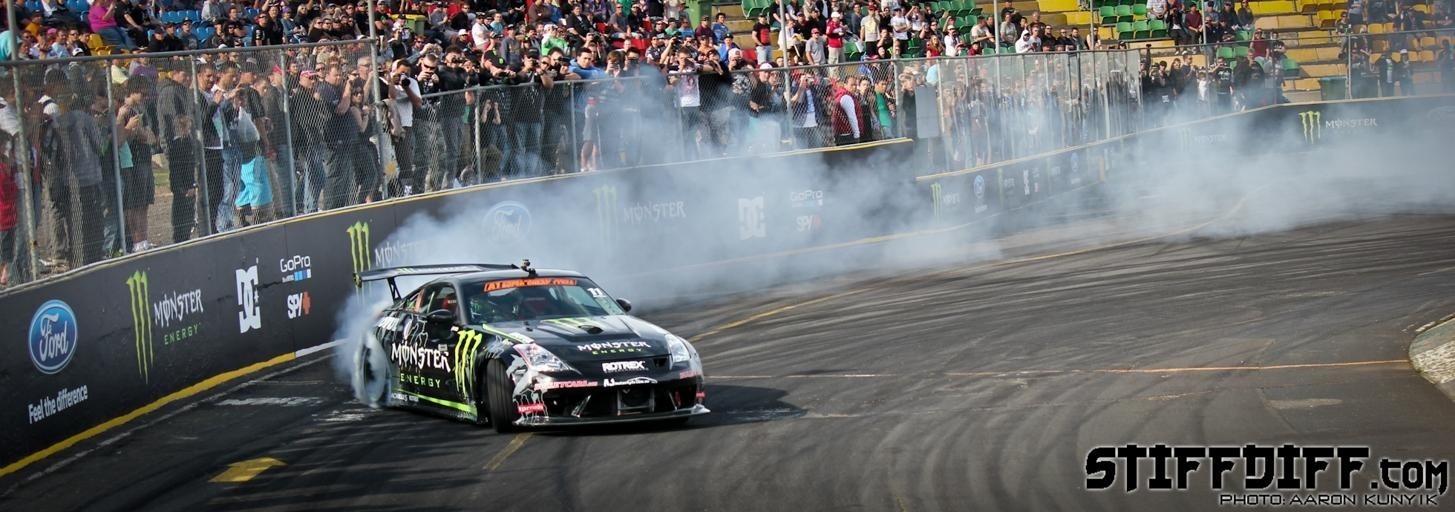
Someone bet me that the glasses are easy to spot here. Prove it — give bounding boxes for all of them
[316,67,325,72]
[424,65,436,69]
[297,2,370,28]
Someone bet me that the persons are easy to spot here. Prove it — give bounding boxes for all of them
[1,2,1455,286]
[454,285,502,323]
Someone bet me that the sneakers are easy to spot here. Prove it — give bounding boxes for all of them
[38,241,159,275]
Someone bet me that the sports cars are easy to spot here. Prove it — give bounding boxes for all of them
[352,260,711,432]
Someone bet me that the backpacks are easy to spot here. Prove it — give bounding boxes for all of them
[28,95,61,165]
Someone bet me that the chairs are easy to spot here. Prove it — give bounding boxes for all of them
[737,0,1035,77]
[1299,0,1455,73]
[1086,0,1301,79]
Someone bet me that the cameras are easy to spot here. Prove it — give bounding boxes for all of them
[590,31,602,44]
[426,71,433,79]
[611,61,624,69]
[566,33,578,46]
[732,57,748,69]
[531,59,541,67]
[672,32,684,52]
[397,73,410,88]
[629,57,639,65]
[499,72,509,77]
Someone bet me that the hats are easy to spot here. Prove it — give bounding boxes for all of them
[476,9,540,69]
[300,68,318,76]
[154,16,192,34]
[46,69,71,86]
[71,47,84,57]
[458,28,469,35]
